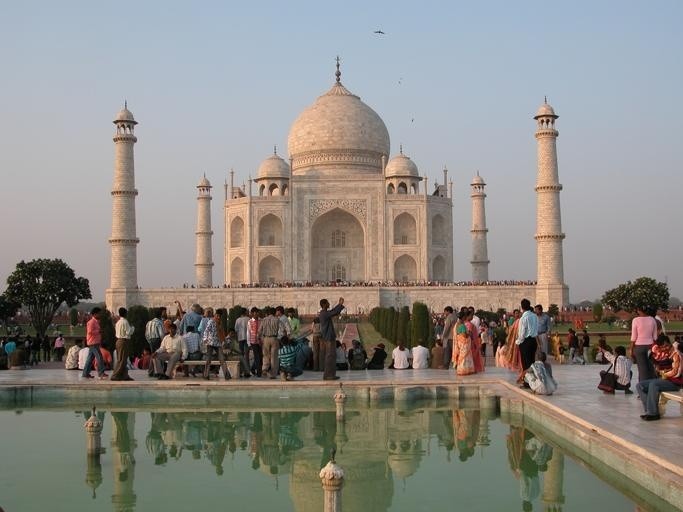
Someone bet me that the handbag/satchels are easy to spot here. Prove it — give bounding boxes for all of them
[597,355,618,392]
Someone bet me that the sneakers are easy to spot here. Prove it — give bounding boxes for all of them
[82,369,340,380]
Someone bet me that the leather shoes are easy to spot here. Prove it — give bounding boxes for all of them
[639,415,644,419]
[643,414,660,421]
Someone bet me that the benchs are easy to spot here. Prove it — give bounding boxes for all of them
[165,360,240,380]
[658,388,683,414]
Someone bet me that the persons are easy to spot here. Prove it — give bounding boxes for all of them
[181,280,538,289]
[562,304,591,313]
[335,339,445,372]
[1,325,66,371]
[568,328,634,394]
[629,304,682,420]
[65,296,344,381]
[441,409,553,512]
[436,298,560,397]
[111,411,336,481]
